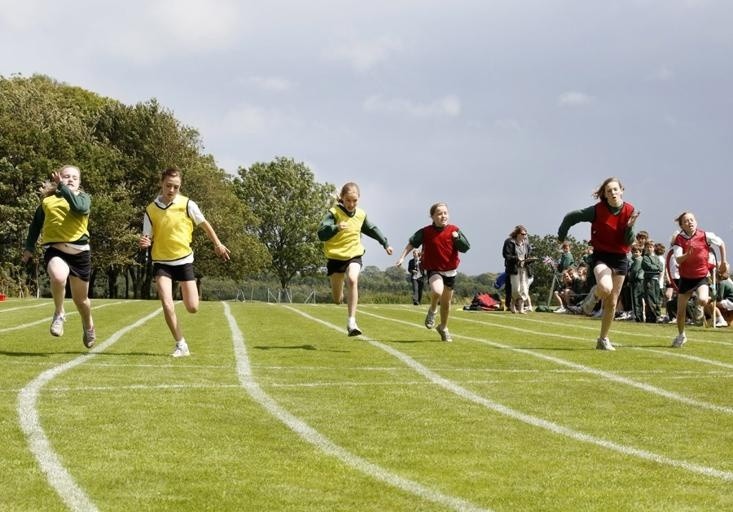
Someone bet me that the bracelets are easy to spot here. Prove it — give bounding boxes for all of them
[214,243,222,248]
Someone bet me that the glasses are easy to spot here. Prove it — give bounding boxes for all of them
[520,233,526,236]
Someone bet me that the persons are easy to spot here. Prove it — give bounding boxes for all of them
[395,201,470,343]
[555,177,642,351]
[407,250,425,306]
[136,167,231,359]
[22,164,97,349]
[500,224,731,329]
[316,182,393,338]
[669,212,728,349]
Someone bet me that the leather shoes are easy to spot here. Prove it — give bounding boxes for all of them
[566,304,582,314]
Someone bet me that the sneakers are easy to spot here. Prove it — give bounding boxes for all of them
[169,343,189,357]
[435,324,452,342]
[671,334,687,348]
[596,338,616,351]
[425,307,437,329]
[615,311,635,321]
[82,322,96,348]
[657,305,729,328]
[553,307,566,313]
[346,323,362,337]
[49,312,65,337]
[583,285,600,315]
